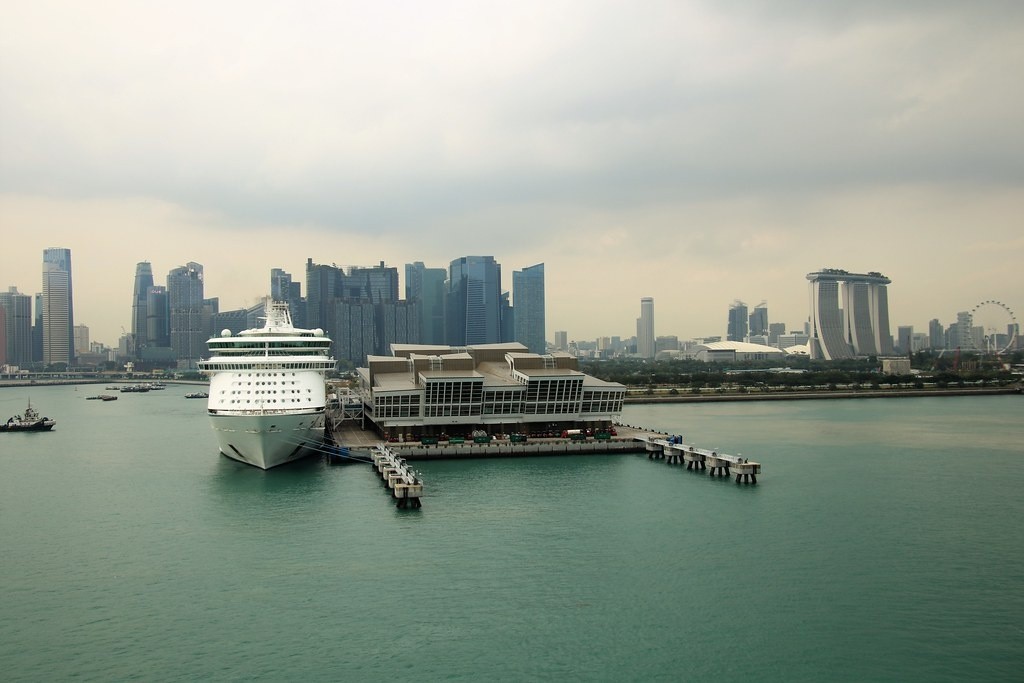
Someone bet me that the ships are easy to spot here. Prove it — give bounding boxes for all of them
[192,296,342,472]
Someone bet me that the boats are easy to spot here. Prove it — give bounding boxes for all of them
[0,398,58,433]
[185,391,209,399]
[105,382,167,392]
